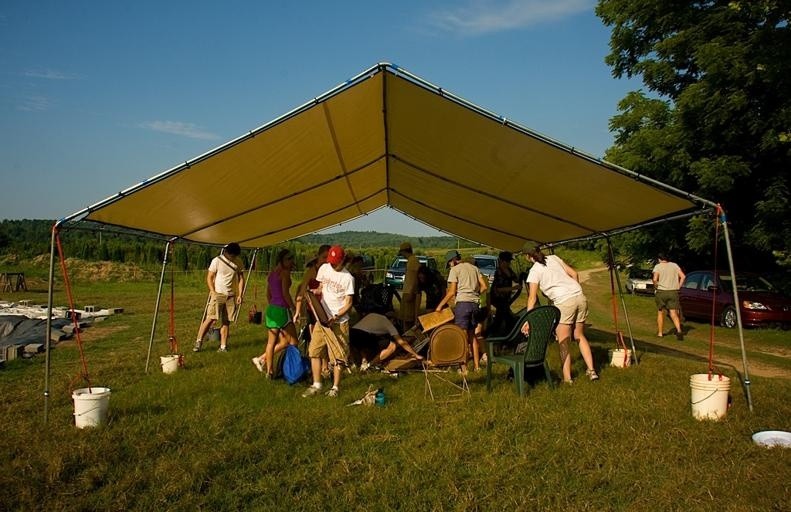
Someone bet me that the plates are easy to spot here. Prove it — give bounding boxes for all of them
[752,430,791,448]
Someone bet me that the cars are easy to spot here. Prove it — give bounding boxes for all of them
[676,270,791,330]
[625,268,656,298]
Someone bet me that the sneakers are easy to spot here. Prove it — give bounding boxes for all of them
[217,347,228,353]
[676,332,683,340]
[457,368,469,375]
[251,357,265,373]
[193,340,202,352]
[586,369,599,381]
[301,385,324,398]
[325,388,340,399]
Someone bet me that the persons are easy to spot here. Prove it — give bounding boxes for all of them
[520,239,601,387]
[300,245,357,399]
[651,251,688,341]
[192,241,246,355]
[250,240,524,380]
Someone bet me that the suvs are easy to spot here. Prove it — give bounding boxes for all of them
[386,254,437,289]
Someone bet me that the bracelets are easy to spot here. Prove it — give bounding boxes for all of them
[335,314,340,319]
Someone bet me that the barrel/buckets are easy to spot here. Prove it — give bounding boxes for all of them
[248,304,260,323]
[689,373,730,423]
[160,353,180,373]
[612,345,632,369]
[208,326,221,340]
[72,386,112,429]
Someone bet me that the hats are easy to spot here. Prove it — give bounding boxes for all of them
[444,250,461,269]
[521,241,540,255]
[326,246,345,265]
[399,242,412,252]
[500,252,515,261]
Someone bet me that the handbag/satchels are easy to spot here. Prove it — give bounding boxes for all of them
[272,344,312,385]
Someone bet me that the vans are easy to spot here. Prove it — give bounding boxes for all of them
[473,254,498,285]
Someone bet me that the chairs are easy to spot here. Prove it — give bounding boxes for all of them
[422,322,474,407]
[484,304,563,396]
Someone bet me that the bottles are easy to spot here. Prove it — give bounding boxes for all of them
[376,388,385,407]
